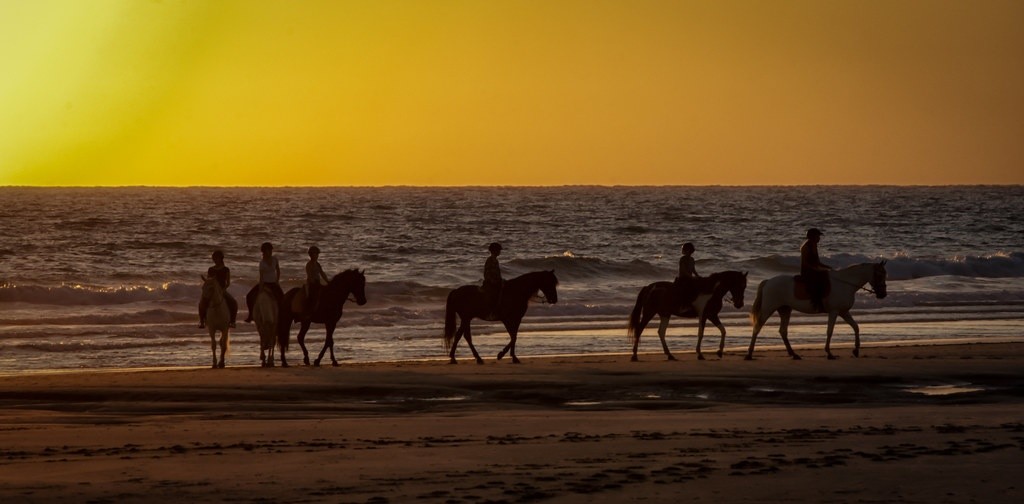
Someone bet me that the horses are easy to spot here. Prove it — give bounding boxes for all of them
[743,258,889,361]
[200,273,230,369]
[251,266,368,368]
[444,270,560,365]
[626,269,750,363]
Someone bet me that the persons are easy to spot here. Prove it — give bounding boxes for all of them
[800,228,832,308]
[246,242,286,329]
[306,245,330,323]
[679,242,703,306]
[198,250,238,328]
[480,242,507,320]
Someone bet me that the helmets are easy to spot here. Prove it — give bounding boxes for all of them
[682,242,695,253]
[261,242,274,251]
[806,228,823,239]
[212,250,224,259]
[489,242,503,250]
[308,246,320,255]
